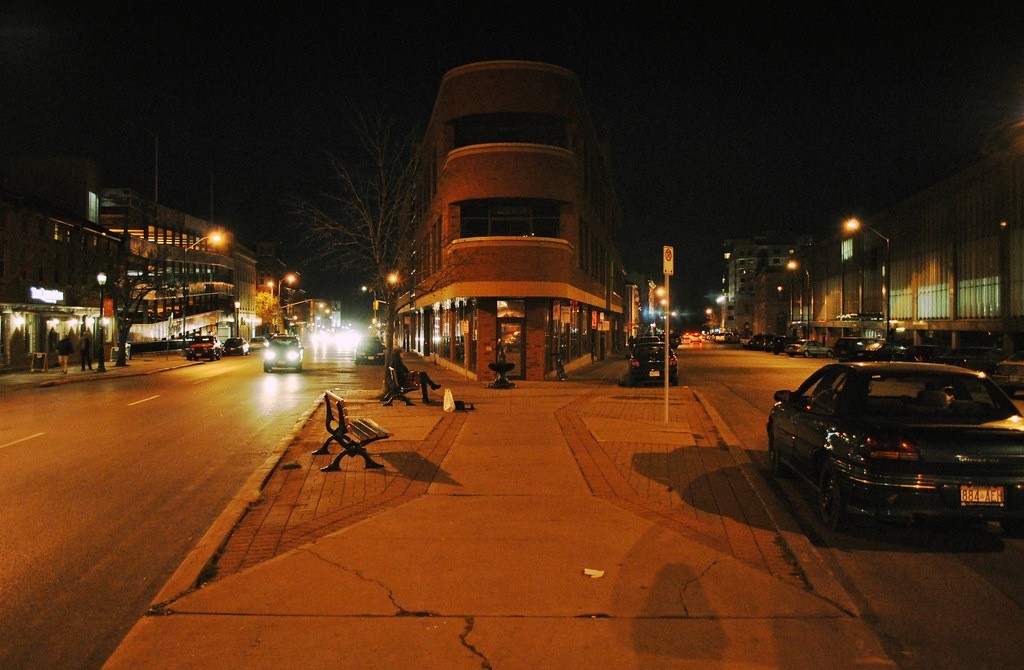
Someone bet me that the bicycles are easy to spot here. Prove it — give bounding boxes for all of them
[550,351,569,382]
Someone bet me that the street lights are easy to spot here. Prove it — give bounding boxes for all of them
[267,274,296,330]
[234,299,240,337]
[180,231,222,358]
[787,258,810,341]
[843,217,891,341]
[97,269,108,372]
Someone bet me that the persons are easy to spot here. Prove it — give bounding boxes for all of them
[392,349,441,404]
[628,336,635,348]
[55,335,74,374]
[925,378,955,407]
[840,372,873,399]
[76,332,95,371]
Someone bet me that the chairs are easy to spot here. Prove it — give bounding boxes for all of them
[914,391,946,411]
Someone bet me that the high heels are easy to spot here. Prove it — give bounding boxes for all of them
[431,383,441,390]
[422,398,431,403]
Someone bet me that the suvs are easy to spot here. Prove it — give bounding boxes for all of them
[263,334,304,373]
[187,335,222,362]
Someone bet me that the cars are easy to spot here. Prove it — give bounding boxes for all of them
[625,327,1024,401]
[221,337,250,356]
[766,360,1024,533]
[249,336,269,353]
[354,338,386,366]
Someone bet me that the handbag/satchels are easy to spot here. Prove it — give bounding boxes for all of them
[443,388,456,412]
[405,370,421,388]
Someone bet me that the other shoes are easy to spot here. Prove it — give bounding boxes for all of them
[64,369,67,374]
[81,368,85,371]
[89,366,94,371]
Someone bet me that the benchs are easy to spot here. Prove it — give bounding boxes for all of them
[313,391,395,472]
[382,366,415,406]
